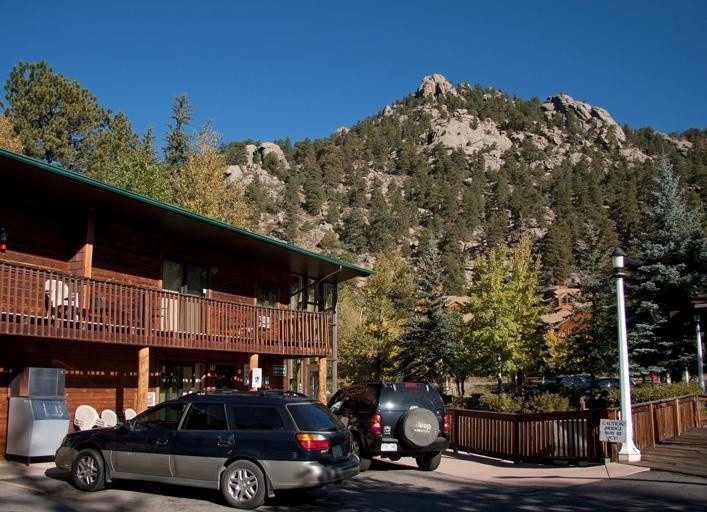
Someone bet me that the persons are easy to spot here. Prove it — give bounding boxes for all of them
[579,389,591,411]
[587,392,608,409]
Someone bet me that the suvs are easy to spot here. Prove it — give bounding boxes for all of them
[326,379,451,472]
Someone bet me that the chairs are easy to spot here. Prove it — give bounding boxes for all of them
[69,401,138,436]
[40,277,80,320]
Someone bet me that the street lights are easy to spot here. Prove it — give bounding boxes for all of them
[607,245,641,462]
[684,363,689,387]
[692,310,704,393]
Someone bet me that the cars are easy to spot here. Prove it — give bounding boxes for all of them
[54,388,360,509]
[540,372,636,393]
